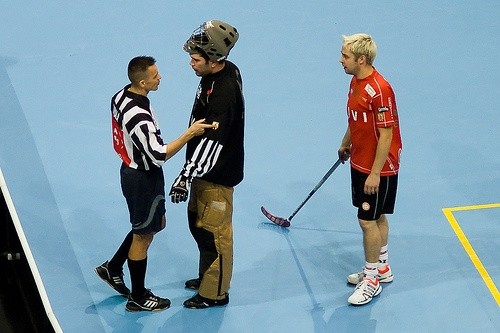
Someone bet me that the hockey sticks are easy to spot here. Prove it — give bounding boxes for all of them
[261,149,350,229]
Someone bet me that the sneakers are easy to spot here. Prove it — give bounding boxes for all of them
[93,260,130,297]
[348,277,383,306]
[185,278,201,289]
[347,263,393,286]
[124,289,170,313]
[183,293,229,309]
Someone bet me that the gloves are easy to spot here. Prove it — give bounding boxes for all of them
[169,171,193,204]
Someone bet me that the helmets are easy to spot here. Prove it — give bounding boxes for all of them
[183,19,239,62]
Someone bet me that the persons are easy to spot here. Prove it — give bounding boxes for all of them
[94,56,218,313]
[337,33,403,305]
[169,19,245,310]
